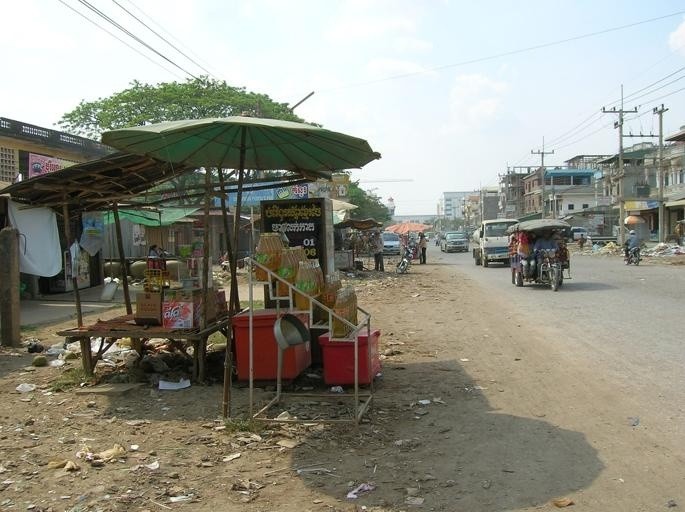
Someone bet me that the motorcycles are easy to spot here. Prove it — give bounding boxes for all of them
[504,219,573,291]
[395,235,423,274]
[620,240,643,266]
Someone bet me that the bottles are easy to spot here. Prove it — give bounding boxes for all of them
[256,233,358,338]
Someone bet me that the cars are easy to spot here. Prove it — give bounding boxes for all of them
[380,232,402,255]
[424,231,469,253]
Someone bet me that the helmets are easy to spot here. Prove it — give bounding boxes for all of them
[630,230,635,234]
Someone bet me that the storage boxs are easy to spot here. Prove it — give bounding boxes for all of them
[232,307,380,386]
[134,288,228,330]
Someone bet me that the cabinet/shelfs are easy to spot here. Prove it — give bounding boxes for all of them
[232,257,381,388]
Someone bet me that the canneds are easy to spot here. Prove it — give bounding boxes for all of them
[147,259,166,275]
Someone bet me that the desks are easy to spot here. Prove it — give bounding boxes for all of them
[55,306,251,384]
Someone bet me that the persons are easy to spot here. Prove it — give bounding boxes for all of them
[370,231,386,271]
[418,231,428,264]
[148,244,159,257]
[509,228,560,281]
[625,229,640,262]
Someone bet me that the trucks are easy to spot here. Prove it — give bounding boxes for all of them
[470,219,520,267]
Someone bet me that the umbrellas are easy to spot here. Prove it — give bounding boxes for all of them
[385,222,433,239]
[102,114,381,420]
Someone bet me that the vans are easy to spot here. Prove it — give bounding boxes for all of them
[561,227,588,243]
